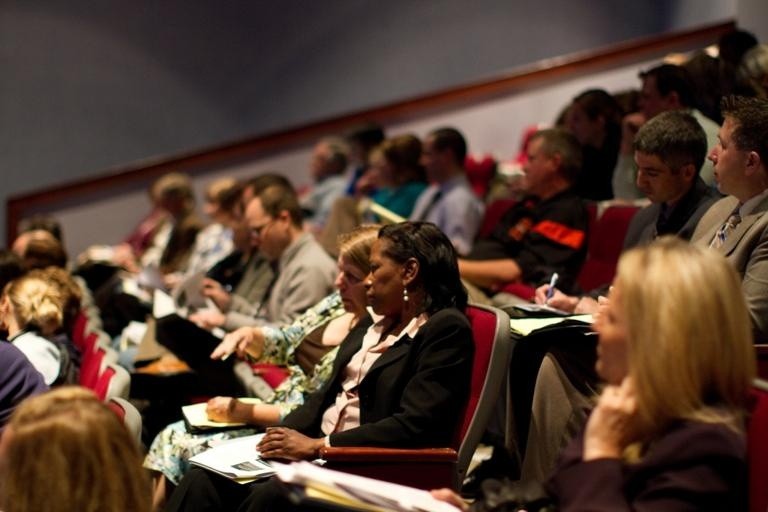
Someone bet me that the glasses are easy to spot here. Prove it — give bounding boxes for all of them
[251,220,270,238]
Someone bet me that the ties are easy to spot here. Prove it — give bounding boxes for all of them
[708,214,741,249]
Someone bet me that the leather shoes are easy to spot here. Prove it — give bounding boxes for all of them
[460,460,520,498]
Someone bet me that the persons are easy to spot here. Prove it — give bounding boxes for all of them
[335,120,386,199]
[0,322,53,436]
[612,63,721,200]
[408,126,485,257]
[230,196,274,303]
[454,129,589,306]
[18,213,63,243]
[361,133,429,223]
[0,269,70,384]
[26,265,82,385]
[110,181,170,273]
[160,176,238,292]
[500,94,768,492]
[1,249,28,294]
[460,108,727,497]
[737,42,768,101]
[297,135,351,240]
[563,88,625,197]
[11,230,63,271]
[713,29,759,97]
[1,385,153,512]
[166,220,476,512]
[241,173,298,314]
[241,234,756,512]
[188,184,340,332]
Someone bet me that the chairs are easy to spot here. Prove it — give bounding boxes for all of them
[464,149,648,299]
[318,304,510,496]
[743,375,768,510]
[68,273,143,447]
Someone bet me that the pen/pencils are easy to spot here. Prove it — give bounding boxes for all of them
[547,273,559,300]
